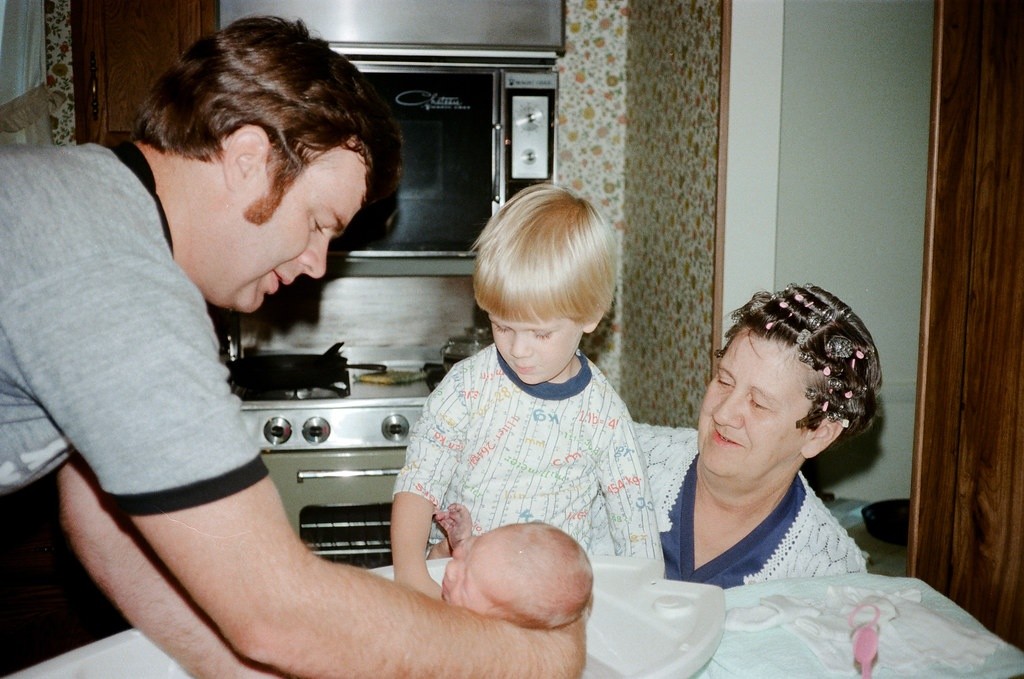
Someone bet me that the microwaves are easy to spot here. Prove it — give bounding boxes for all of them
[310,44,565,277]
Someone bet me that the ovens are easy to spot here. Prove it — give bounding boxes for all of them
[260,448,437,577]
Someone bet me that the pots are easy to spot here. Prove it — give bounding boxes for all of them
[442,323,493,371]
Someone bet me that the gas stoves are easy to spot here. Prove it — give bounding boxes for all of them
[222,349,444,454]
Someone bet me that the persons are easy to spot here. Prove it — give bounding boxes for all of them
[385,184,664,600]
[430,498,597,629]
[631,282,885,583]
[2,15,586,679]
